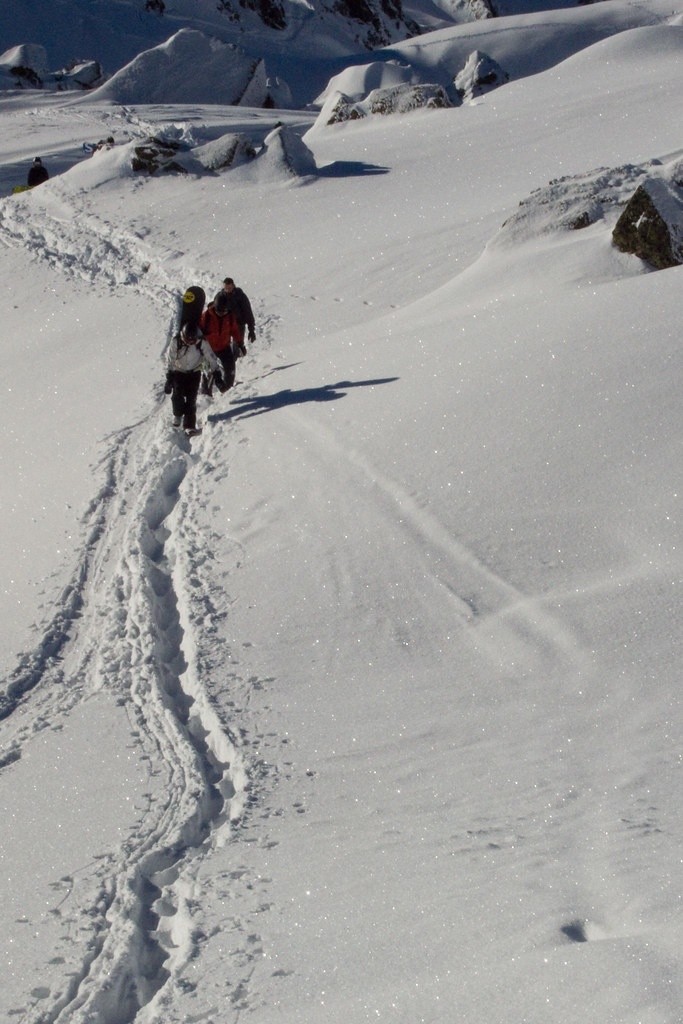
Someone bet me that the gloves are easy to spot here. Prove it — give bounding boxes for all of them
[248,328,256,342]
[214,371,226,392]
[239,341,247,355]
[164,374,175,394]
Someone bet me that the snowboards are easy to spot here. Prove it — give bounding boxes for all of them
[181,285,206,330]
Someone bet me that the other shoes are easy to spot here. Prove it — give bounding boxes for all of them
[174,416,182,427]
[184,428,197,436]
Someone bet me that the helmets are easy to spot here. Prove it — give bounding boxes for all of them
[33,157,42,165]
[182,324,198,339]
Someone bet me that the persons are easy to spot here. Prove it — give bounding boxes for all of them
[27,157,49,186]
[219,277,256,357]
[107,137,114,143]
[163,323,225,437]
[201,295,247,397]
